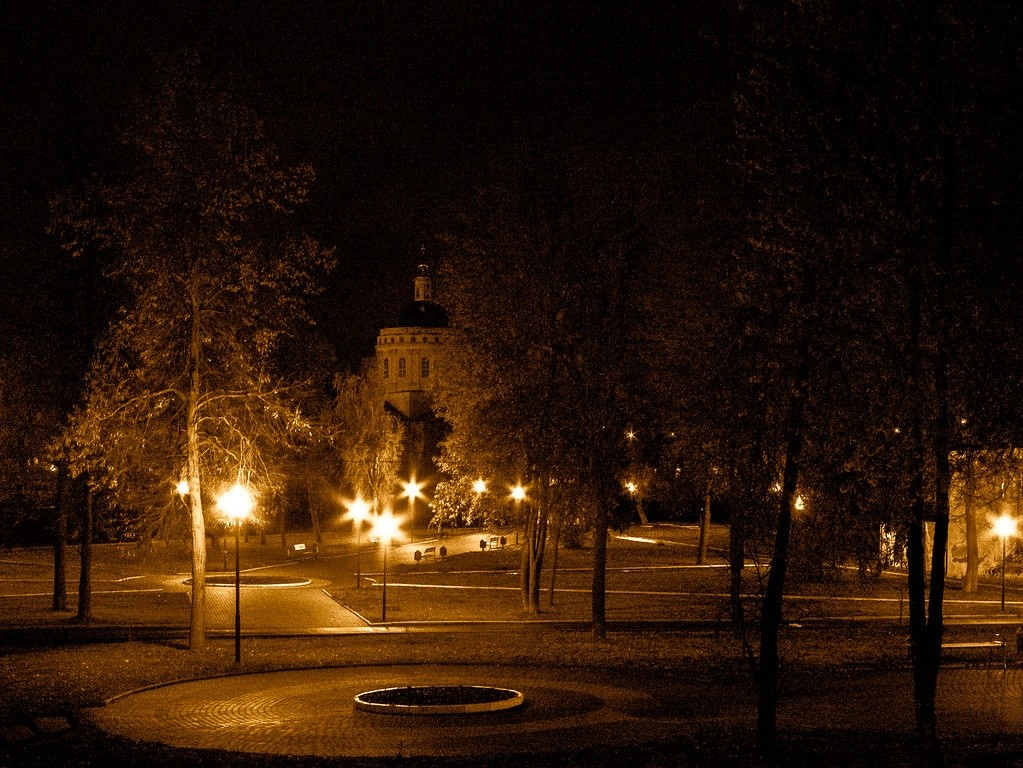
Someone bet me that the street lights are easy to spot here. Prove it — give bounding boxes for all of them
[512,487,524,545]
[351,498,371,588]
[223,486,252,663]
[374,511,397,622]
[405,482,418,541]
[995,515,1017,611]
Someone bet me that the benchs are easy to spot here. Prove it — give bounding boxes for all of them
[905,633,1008,676]
